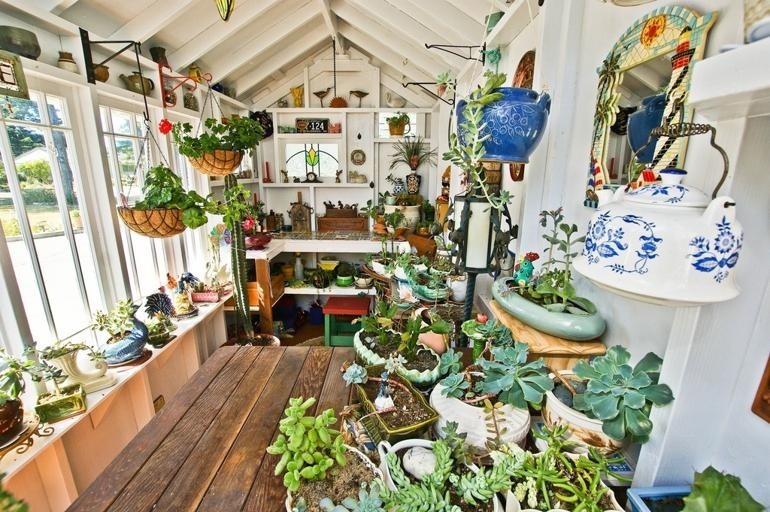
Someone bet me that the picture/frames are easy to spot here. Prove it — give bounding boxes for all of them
[0,51,29,101]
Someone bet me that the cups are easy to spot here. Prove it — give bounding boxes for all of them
[291,88,304,108]
[148,47,168,66]
[93,63,109,83]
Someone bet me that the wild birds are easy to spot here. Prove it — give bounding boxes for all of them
[312,86,334,108]
[348,90,370,107]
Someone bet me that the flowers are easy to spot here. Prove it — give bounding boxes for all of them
[159,116,268,158]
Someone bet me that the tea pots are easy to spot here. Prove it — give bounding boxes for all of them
[455,87,550,163]
[119,72,155,97]
[572,121,744,302]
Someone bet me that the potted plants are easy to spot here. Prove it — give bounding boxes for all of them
[43,342,119,394]
[159,274,199,319]
[4,344,87,426]
[94,301,148,366]
[147,324,176,349]
[144,294,179,331]
[265,58,677,512]
[215,174,282,347]
[116,167,208,239]
[2,356,55,456]
[180,273,220,303]
[624,464,769,512]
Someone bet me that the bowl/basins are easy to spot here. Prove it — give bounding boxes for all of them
[244,233,272,250]
[0,26,41,59]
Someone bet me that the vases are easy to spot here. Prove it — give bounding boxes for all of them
[182,145,245,176]
[150,45,170,68]
[57,50,79,73]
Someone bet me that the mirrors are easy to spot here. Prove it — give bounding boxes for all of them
[580,7,723,209]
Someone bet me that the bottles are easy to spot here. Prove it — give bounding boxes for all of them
[393,179,405,195]
[188,63,201,83]
[213,83,224,93]
[57,51,79,74]
[185,92,197,110]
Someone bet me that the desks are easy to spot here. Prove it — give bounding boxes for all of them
[65,344,521,512]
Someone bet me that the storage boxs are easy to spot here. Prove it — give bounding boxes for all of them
[225,274,282,307]
